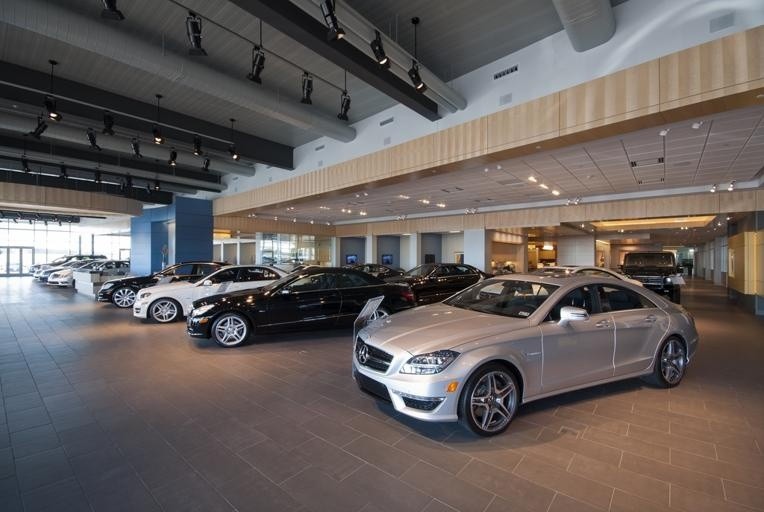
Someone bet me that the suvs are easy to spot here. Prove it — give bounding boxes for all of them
[616,250,683,304]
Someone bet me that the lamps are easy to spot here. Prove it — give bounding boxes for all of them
[17,108,212,173]
[301,0,427,96]
[89,1,350,127]
[31,60,241,162]
[13,149,162,199]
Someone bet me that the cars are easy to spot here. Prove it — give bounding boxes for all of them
[185,267,417,348]
[379,263,496,306]
[261,253,314,265]
[98,259,233,307]
[351,273,699,437]
[339,263,405,280]
[133,269,294,324]
[513,265,642,296]
[4,254,129,289]
